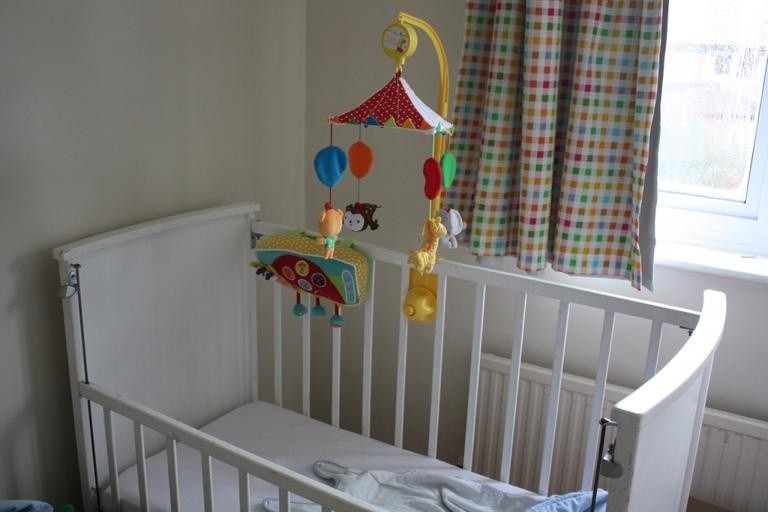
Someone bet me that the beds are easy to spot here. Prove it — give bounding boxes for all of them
[52,200,726,512]
